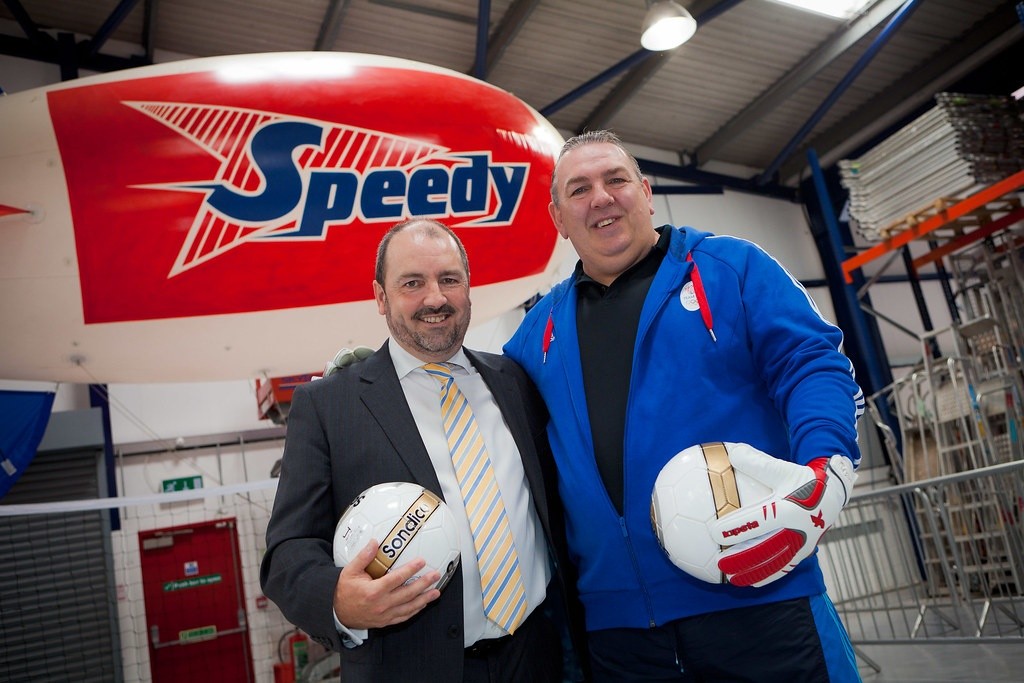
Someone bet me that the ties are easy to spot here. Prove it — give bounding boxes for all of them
[420,362,529,635]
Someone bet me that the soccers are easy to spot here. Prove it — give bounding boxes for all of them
[646,441,789,587]
[331,482,462,599]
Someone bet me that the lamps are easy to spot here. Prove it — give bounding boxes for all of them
[640,0,697,52]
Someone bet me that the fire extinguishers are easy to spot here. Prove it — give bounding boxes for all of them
[277,626,310,683]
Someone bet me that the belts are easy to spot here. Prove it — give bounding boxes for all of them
[464,579,553,659]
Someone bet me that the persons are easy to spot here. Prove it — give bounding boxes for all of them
[259,216,617,683]
[322,132,867,683]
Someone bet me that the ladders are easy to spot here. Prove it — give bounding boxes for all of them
[919,313,1023,612]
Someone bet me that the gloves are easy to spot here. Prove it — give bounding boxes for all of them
[709,442,859,588]
[310,347,376,381]
